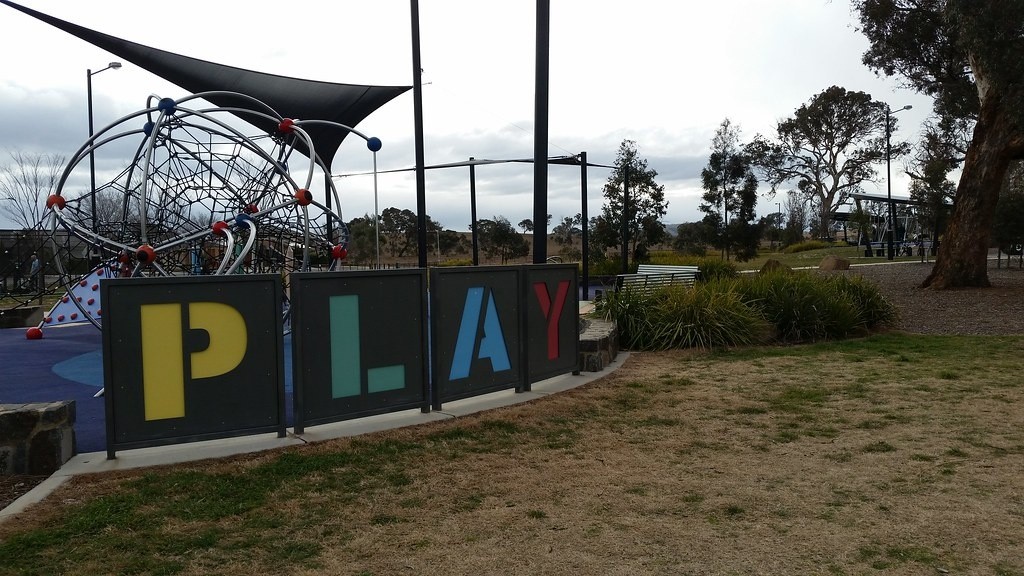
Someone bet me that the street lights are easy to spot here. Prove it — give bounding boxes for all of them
[88,62,122,264]
[435,230,439,262]
[887,104,913,260]
[775,202,780,231]
[13,231,21,291]
